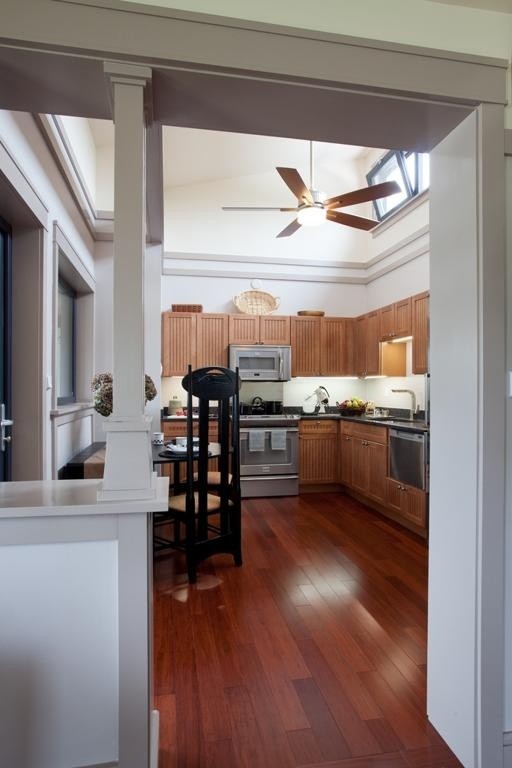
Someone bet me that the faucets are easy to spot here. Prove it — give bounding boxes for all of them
[391,389,417,420]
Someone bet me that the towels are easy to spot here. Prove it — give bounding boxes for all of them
[270,429,287,450]
[248,429,265,451]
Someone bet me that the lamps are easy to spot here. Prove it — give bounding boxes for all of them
[293,206,328,228]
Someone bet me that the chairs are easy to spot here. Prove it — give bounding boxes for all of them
[155,364,245,584]
[173,363,244,543]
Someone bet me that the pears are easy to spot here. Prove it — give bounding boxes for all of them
[347,398,365,408]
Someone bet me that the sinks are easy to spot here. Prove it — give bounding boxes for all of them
[367,416,419,423]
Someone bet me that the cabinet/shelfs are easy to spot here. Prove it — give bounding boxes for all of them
[229,313,291,344]
[161,311,229,377]
[291,316,352,377]
[300,419,340,493]
[412,290,430,374]
[161,421,232,486]
[387,476,429,539]
[340,419,387,517]
[353,309,407,378]
[379,296,412,340]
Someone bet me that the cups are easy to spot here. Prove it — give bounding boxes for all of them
[239,403,249,414]
[373,409,389,417]
[176,436,188,447]
[153,432,165,444]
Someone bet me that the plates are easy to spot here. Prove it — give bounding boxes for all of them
[297,310,324,316]
[165,447,200,455]
[193,436,200,442]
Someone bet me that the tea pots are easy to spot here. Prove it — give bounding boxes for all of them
[250,396,265,415]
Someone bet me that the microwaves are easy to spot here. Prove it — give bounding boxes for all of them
[229,344,291,382]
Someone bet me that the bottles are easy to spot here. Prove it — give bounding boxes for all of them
[167,398,184,415]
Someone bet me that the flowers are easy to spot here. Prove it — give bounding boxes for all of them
[89,371,157,417]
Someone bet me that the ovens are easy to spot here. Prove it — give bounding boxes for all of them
[239,427,300,498]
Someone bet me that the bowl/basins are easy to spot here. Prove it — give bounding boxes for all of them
[337,406,365,416]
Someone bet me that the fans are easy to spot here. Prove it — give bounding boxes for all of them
[221,139,403,239]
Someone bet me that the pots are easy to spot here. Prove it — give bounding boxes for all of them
[265,400,283,414]
